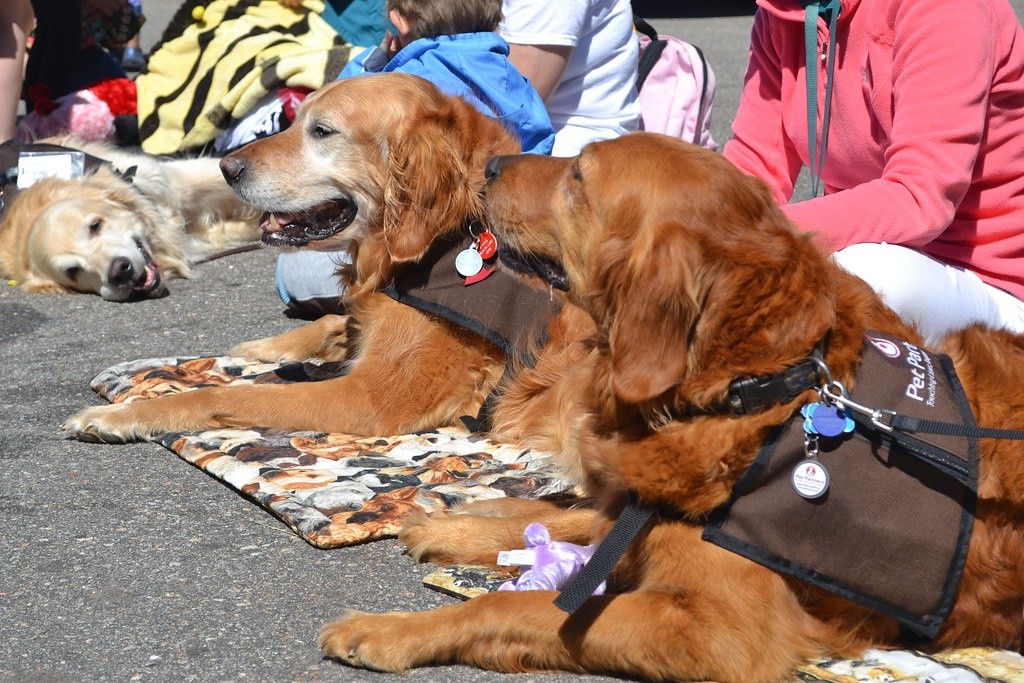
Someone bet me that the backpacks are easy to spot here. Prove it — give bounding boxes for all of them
[631,13,721,151]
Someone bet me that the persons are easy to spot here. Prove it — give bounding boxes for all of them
[721,0,1024,345]
[1,0,148,141]
[490,1,640,163]
[332,0,557,159]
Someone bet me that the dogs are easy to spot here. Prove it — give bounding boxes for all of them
[314,130,1024,683]
[60,72,599,458]
[1,130,269,305]
